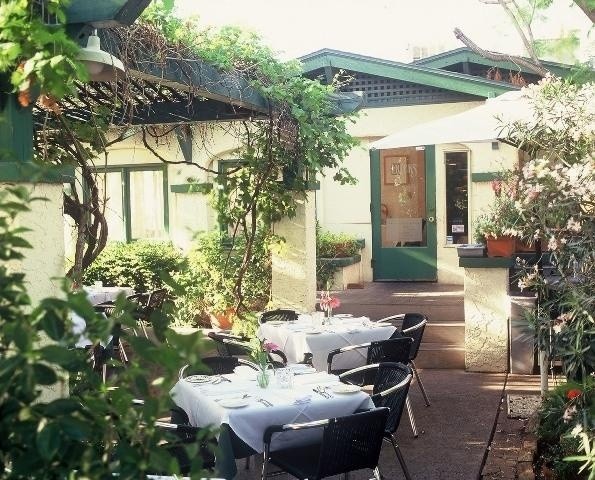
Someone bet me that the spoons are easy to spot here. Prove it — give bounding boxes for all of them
[316,385,333,398]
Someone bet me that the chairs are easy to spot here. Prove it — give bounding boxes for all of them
[75,288,431,480]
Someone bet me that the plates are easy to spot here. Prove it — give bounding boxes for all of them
[184,374,212,383]
[268,321,393,335]
[334,314,352,318]
[333,385,360,393]
[219,398,249,408]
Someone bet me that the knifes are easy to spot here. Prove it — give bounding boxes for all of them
[313,389,329,399]
[215,373,231,382]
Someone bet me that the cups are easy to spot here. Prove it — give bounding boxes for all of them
[95,281,102,294]
[274,367,294,389]
[297,312,325,332]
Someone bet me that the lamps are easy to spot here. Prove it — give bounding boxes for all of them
[75,28,126,82]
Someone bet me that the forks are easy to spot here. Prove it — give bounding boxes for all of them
[257,398,273,407]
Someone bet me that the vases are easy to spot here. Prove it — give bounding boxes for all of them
[485,235,514,259]
[514,234,538,252]
[331,244,352,259]
